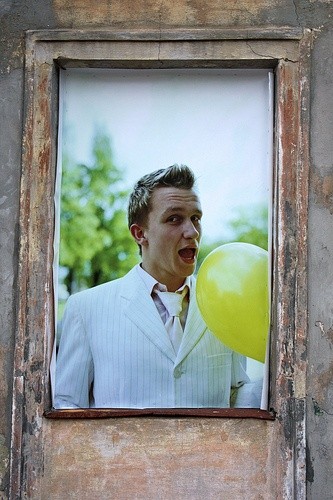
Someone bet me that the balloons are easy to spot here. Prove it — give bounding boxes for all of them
[195,242,269,364]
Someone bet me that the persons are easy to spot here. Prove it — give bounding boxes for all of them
[54,165,265,409]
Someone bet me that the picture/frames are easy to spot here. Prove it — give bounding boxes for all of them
[42,56,278,418]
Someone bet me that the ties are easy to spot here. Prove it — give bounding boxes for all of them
[152,285,189,358]
